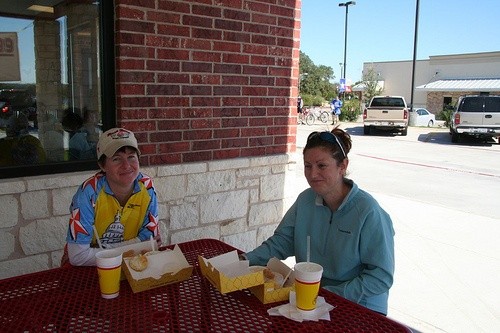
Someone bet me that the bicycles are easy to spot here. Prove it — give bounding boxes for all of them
[305,109,330,126]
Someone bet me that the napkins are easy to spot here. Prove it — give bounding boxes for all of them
[267,291,336,322]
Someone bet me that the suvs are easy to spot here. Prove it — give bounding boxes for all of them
[445,92,500,145]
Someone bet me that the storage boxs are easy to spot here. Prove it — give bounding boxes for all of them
[108,240,193,294]
[198,250,293,305]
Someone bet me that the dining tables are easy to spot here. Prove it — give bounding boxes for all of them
[0,238,412,333]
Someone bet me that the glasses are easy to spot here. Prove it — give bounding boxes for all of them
[307,132,346,156]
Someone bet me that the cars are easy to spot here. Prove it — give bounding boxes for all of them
[407,107,436,128]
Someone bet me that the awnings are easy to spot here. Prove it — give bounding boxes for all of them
[416,77,500,92]
[351,81,385,93]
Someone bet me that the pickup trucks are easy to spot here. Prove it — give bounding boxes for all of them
[362,95,409,137]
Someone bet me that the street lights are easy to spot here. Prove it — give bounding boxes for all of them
[298,72,308,92]
[339,62,343,79]
[338,0,356,107]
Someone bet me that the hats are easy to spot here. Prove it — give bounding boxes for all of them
[97,128,141,160]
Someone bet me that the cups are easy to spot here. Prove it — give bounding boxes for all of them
[95,249,123,299]
[294,262,324,312]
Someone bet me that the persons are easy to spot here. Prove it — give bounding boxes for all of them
[240,125,396,333]
[57,127,162,332]
[0,100,102,169]
[330,96,342,126]
[297,95,306,125]
